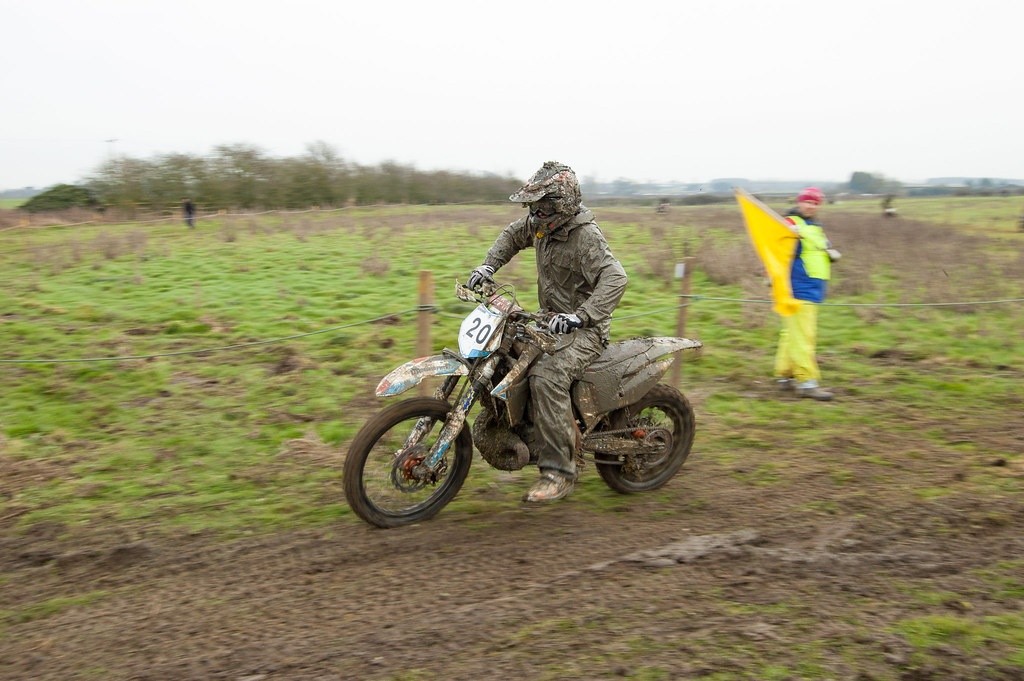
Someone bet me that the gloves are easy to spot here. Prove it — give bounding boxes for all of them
[466,265,495,291]
[548,313,585,334]
[826,249,841,259]
[791,225,800,231]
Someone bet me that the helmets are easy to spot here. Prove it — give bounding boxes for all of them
[509,161,582,239]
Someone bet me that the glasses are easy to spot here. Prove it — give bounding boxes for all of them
[530,198,556,216]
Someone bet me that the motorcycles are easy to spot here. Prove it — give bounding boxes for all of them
[343,275,703,528]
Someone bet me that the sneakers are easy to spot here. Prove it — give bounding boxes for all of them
[523,470,574,503]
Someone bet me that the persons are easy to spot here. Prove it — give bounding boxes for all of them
[180,196,197,230]
[467,161,629,505]
[773,188,841,400]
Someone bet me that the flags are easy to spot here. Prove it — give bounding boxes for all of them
[735,186,802,317]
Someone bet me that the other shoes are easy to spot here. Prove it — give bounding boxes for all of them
[795,388,834,401]
[778,382,794,390]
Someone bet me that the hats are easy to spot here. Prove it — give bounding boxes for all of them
[797,187,823,204]
